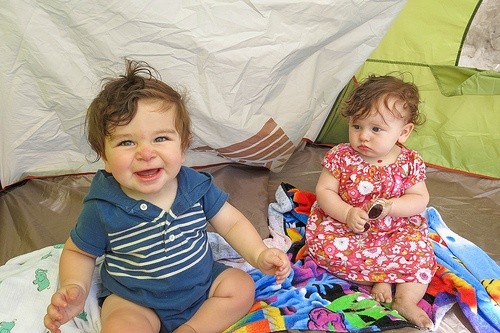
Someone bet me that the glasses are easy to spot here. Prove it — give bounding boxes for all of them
[364,200,386,232]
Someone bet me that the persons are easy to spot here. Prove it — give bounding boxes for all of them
[44,60,291,333]
[305,71,436,330]
